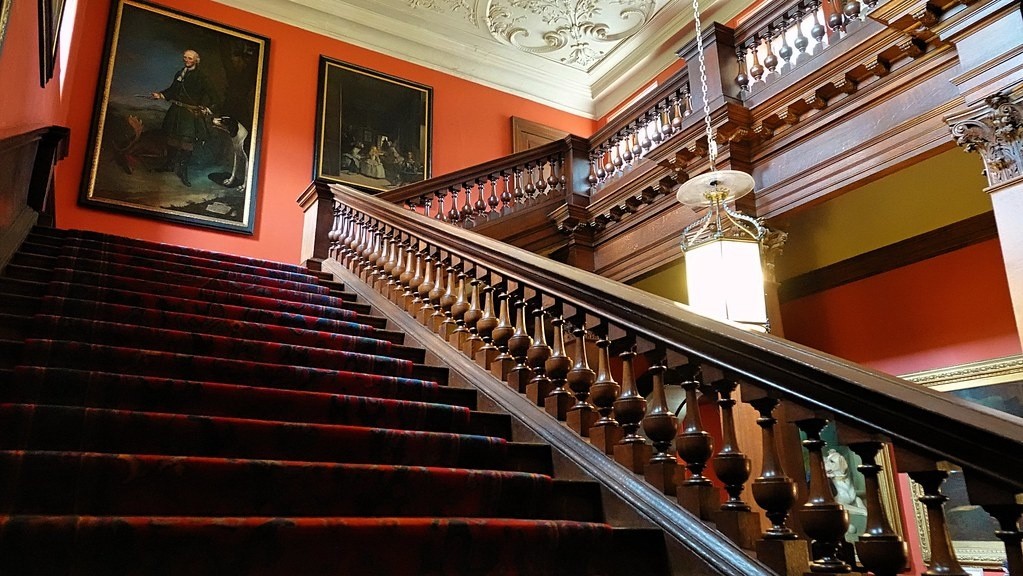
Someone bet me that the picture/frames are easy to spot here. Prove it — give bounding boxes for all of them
[312,54,432,205]
[77,0,271,235]
[792,417,905,557]
[896,353,1023,572]
[39,0,66,86]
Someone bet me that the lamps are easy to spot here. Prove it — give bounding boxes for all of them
[676,0,771,335]
